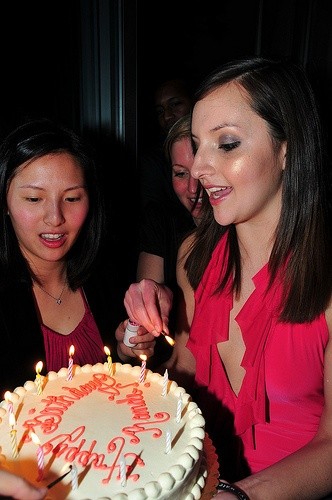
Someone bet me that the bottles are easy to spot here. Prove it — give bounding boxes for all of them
[123,319,140,348]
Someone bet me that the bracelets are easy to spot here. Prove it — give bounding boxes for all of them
[217,482,250,500]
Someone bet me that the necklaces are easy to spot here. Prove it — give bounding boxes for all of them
[40,281,66,305]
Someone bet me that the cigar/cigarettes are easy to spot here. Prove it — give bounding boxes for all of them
[160,330,176,347]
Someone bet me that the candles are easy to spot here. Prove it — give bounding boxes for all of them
[67,344,75,380]
[104,345,114,376]
[176,392,182,422]
[166,427,172,453]
[162,369,169,396]
[120,453,126,484]
[140,354,147,382]
[71,462,79,491]
[31,433,45,480]
[9,412,20,456]
[35,360,43,395]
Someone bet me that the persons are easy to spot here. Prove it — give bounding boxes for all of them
[153,56,332,500]
[118,62,204,373]
[0,116,156,500]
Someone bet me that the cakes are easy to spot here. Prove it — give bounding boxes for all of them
[0,362,208,500]
